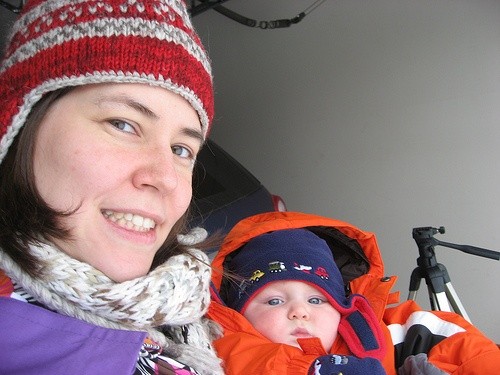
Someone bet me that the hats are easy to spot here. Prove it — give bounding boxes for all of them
[219,229,384,357]
[0,0,216,165]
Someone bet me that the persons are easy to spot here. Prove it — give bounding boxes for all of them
[1,0,225,375]
[202,211,500,375]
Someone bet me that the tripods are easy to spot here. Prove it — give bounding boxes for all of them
[408,227,500,326]
